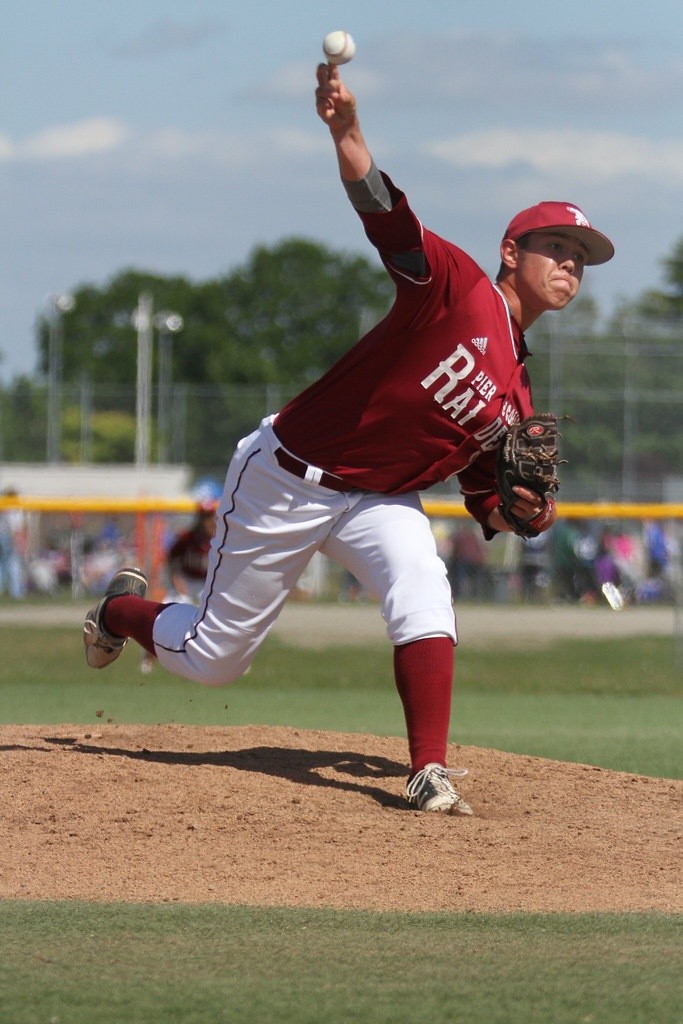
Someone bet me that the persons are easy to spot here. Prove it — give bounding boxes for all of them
[81,61,617,813]
[0,487,188,601]
[138,500,217,673]
[432,515,670,609]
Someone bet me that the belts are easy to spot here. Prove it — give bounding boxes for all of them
[275,448,354,493]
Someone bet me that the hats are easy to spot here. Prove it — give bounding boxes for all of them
[500,202,614,266]
[195,500,218,517]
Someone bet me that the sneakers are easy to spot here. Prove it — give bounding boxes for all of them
[406,763,473,817]
[83,568,149,669]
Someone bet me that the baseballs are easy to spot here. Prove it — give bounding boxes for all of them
[322,30,356,65]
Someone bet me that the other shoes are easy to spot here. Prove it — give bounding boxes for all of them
[142,653,154,674]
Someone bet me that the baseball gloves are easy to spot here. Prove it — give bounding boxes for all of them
[495,412,577,543]
